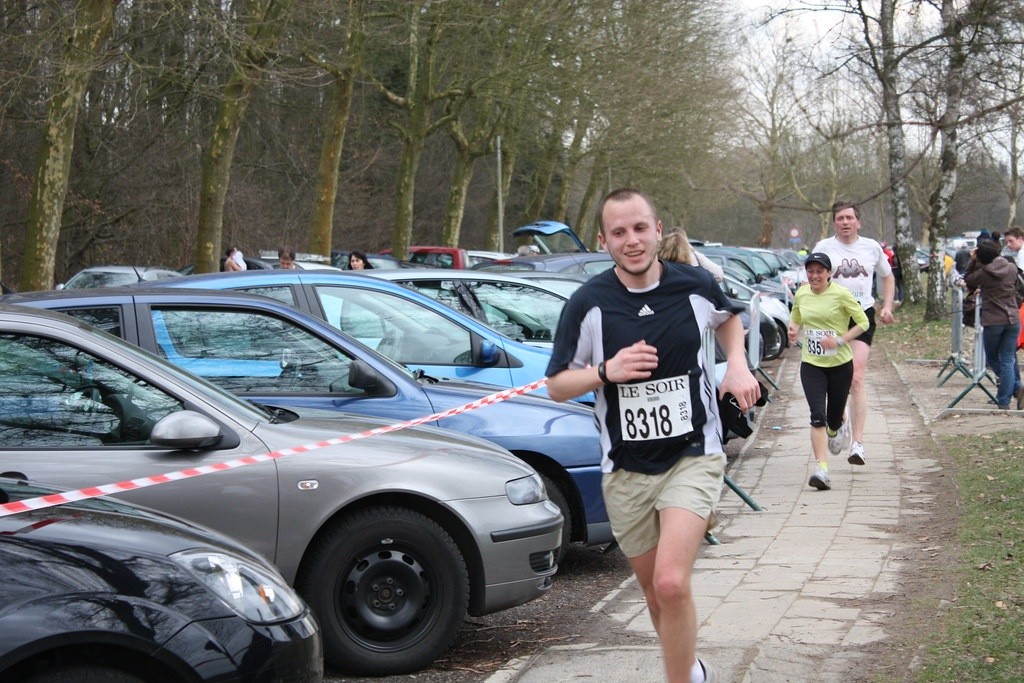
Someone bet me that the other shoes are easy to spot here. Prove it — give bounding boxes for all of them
[988,394,998,404]
[692,658,717,683]
[1017,386,1024,410]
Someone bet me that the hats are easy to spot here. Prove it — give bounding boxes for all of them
[805,253,831,270]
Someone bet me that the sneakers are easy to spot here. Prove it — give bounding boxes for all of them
[840,407,850,451]
[847,442,865,465]
[808,469,831,490]
[827,426,842,455]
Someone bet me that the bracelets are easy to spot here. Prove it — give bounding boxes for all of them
[836,337,844,346]
[598,362,611,385]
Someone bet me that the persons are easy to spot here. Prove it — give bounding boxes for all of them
[787,253,870,491]
[658,227,724,283]
[952,226,1024,411]
[223,246,247,272]
[514,244,540,258]
[545,189,761,683]
[880,241,904,308]
[799,244,809,256]
[278,246,299,270]
[348,250,373,270]
[813,203,895,466]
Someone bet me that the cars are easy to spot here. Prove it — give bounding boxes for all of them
[55,265,183,290]
[0,289,616,563]
[125,268,597,409]
[0,300,566,678]
[180,219,811,392]
[887,230,982,272]
[0,477,326,683]
